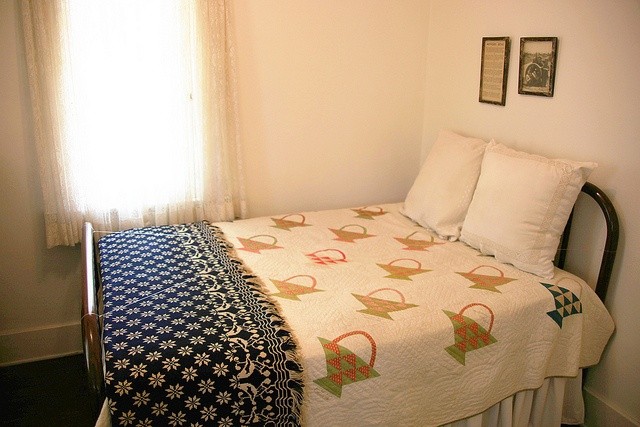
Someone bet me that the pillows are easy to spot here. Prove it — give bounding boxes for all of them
[457,138,602,281]
[398,132,489,243]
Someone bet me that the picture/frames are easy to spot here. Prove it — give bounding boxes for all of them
[516,35,557,97]
[480,37,510,106]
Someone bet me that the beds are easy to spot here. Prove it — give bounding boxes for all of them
[81,183,619,427]
